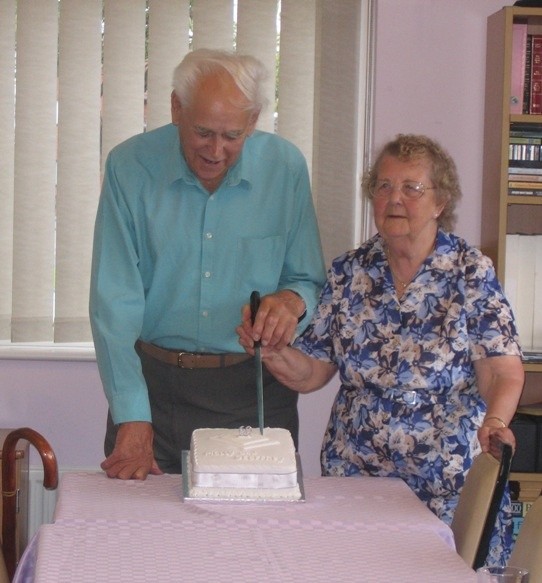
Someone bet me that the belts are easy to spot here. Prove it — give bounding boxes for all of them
[138,341,253,368]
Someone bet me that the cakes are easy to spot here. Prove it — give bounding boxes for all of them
[186,427,302,501]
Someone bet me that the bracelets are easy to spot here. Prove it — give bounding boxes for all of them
[482,416,506,428]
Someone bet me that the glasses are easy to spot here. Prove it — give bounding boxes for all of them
[368,178,438,200]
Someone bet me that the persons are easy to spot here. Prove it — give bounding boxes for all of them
[88,47,328,481]
[233,131,525,577]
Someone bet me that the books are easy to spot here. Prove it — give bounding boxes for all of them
[507,123,542,198]
[509,23,542,115]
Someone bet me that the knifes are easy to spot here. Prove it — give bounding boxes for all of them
[250,290,264,435]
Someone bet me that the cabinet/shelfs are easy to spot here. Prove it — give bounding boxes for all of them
[480,6,542,373]
[1,428,30,583]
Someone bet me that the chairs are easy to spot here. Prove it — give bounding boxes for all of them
[450,434,511,570]
[501,488,542,582]
